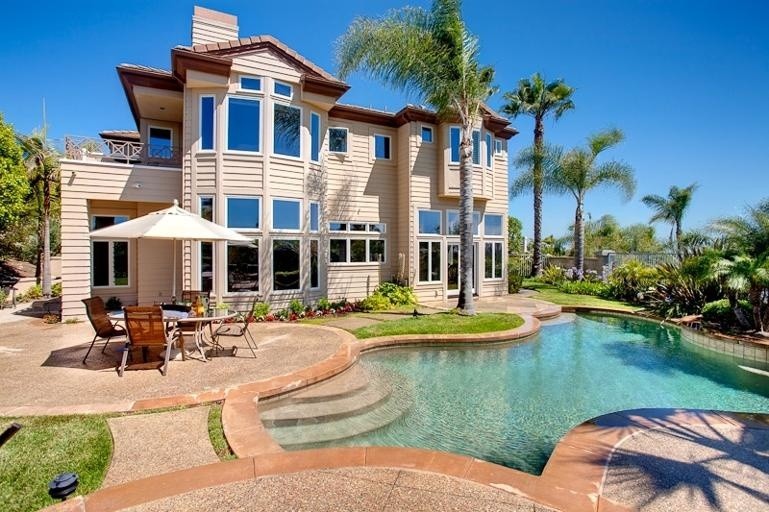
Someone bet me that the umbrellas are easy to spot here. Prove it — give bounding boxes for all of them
[89,197,260,306]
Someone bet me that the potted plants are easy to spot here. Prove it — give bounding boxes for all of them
[215,303,230,317]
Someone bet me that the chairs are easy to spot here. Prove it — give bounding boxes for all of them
[80,296,127,364]
[182,289,224,351]
[118,306,185,375]
[215,298,260,359]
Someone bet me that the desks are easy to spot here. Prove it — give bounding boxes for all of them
[106,307,238,362]
